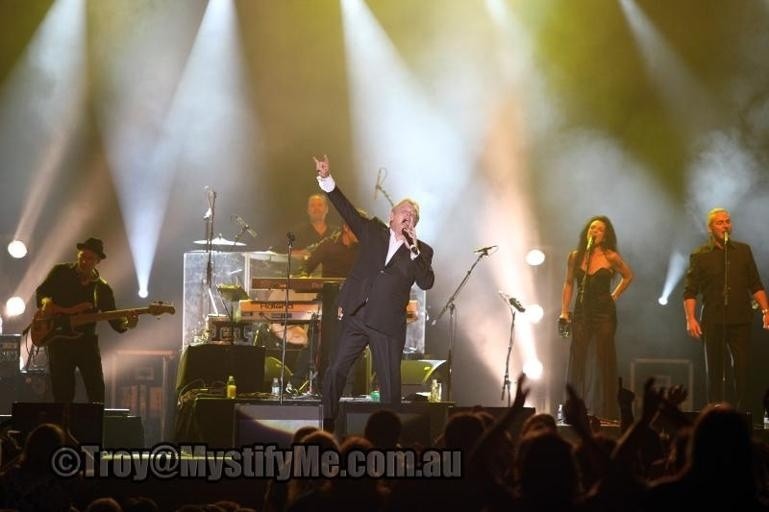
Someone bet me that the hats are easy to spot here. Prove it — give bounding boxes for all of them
[75,236,106,261]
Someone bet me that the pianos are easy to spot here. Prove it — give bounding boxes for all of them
[253,277,348,293]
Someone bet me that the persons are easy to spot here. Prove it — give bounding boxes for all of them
[2,371,769,510]
[308,152,436,425]
[35,236,140,403]
[291,194,338,252]
[556,215,635,425]
[681,206,769,411]
[301,207,367,399]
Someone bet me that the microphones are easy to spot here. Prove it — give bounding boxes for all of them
[401,227,419,256]
[234,215,257,237]
[374,169,381,201]
[474,245,492,253]
[585,234,595,251]
[286,231,296,242]
[509,297,525,313]
[723,232,729,246]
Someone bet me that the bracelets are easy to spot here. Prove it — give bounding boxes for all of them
[762,306,769,315]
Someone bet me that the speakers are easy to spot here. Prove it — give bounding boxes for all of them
[12,401,145,453]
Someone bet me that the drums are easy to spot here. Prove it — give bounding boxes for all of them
[209,316,252,346]
[265,321,308,367]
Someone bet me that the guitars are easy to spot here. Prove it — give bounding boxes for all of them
[31,303,175,347]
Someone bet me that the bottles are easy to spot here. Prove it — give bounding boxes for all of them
[431,376,442,403]
[226,375,237,399]
[271,377,281,395]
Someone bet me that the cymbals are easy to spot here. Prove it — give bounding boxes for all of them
[244,250,289,263]
[195,238,245,247]
